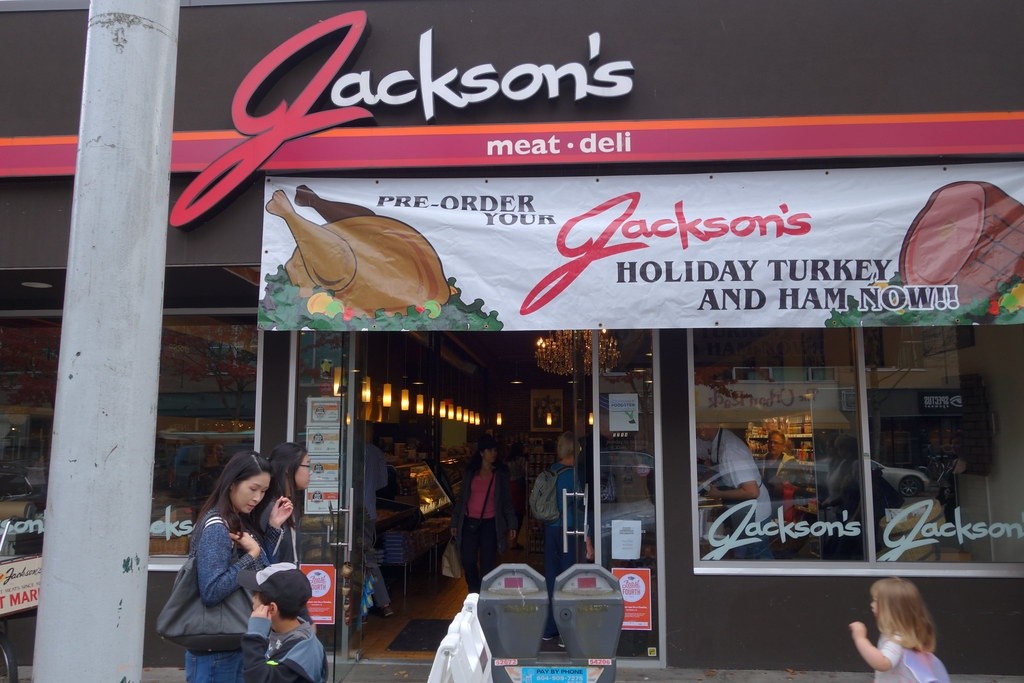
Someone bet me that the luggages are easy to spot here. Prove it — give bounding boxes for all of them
[818,504,857,563]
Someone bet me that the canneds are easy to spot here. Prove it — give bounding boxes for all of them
[528,454,549,478]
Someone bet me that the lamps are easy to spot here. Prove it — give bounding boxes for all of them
[534,330,621,379]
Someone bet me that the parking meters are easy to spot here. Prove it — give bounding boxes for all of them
[474,562,626,683]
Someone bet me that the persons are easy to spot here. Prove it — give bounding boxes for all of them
[758,430,808,560]
[944,429,991,527]
[182,438,227,557]
[696,407,772,561]
[849,578,950,683]
[154,451,294,683]
[451,436,517,594]
[366,423,387,549]
[819,433,878,561]
[259,443,313,562]
[542,432,594,648]
[236,562,329,683]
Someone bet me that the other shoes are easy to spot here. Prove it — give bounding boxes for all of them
[380,606,393,615]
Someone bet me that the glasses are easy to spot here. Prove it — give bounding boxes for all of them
[767,440,784,446]
[296,464,313,471]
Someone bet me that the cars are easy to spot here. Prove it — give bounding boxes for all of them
[697,459,827,559]
[599,449,657,504]
[867,456,930,499]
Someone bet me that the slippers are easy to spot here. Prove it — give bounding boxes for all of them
[510,543,524,550]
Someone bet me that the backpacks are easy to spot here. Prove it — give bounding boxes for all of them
[528,463,577,522]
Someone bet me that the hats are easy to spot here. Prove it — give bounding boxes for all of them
[239,562,312,614]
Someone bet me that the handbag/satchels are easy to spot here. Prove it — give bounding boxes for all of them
[847,469,906,522]
[462,517,483,536]
[441,532,463,579]
[155,513,254,654]
[600,468,616,502]
